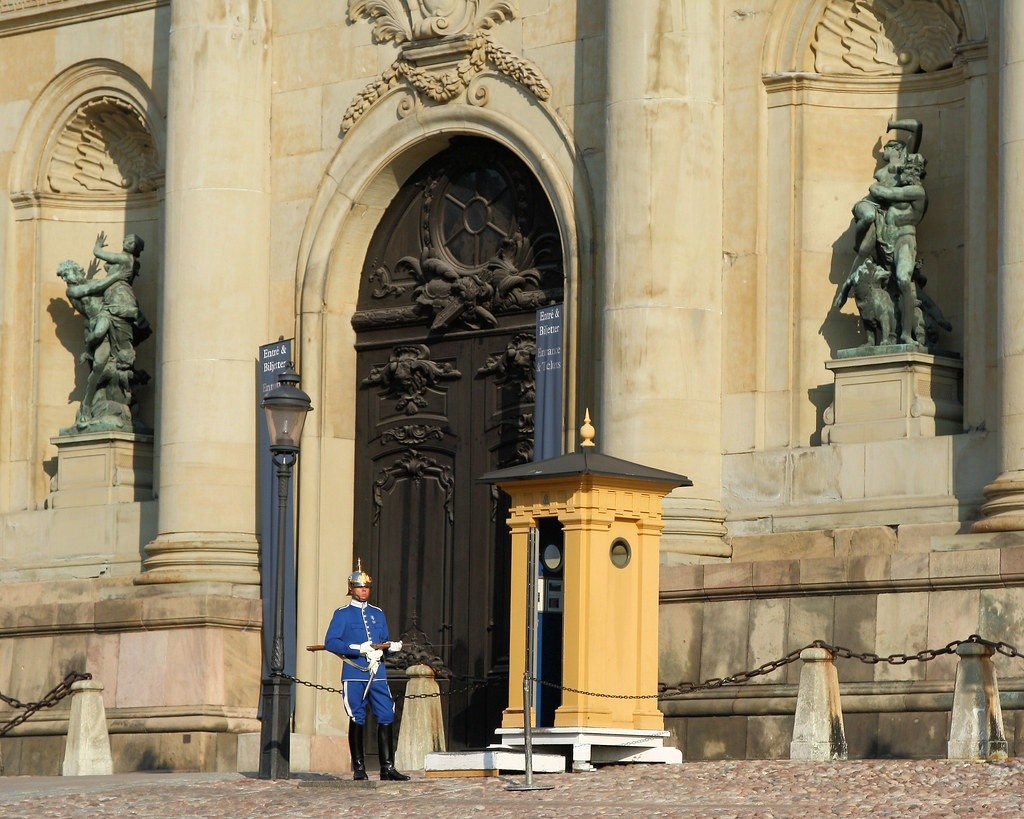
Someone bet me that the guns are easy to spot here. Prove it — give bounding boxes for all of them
[305,644,469,651]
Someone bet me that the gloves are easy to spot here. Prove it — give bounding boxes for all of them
[387,640,403,654]
[361,640,375,653]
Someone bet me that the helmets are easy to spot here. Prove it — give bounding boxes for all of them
[348,571,372,587]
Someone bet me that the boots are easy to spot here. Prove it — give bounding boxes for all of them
[348,719,369,779]
[378,723,411,781]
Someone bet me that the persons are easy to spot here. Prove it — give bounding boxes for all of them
[833,114,953,345]
[57,230,152,423]
[325,569,413,780]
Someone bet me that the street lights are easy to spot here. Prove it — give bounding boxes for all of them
[257,359,315,782]
